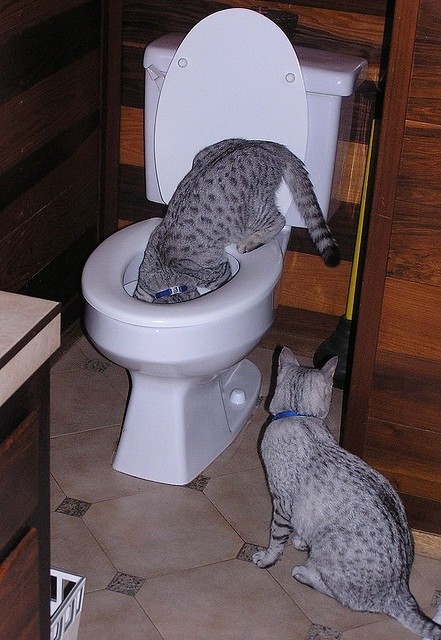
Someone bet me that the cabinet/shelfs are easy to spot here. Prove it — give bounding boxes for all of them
[0,364,52,638]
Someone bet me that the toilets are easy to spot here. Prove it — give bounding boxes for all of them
[81,6,370,488]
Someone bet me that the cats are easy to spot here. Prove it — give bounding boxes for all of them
[250,346,441,640]
[133,136,343,305]
